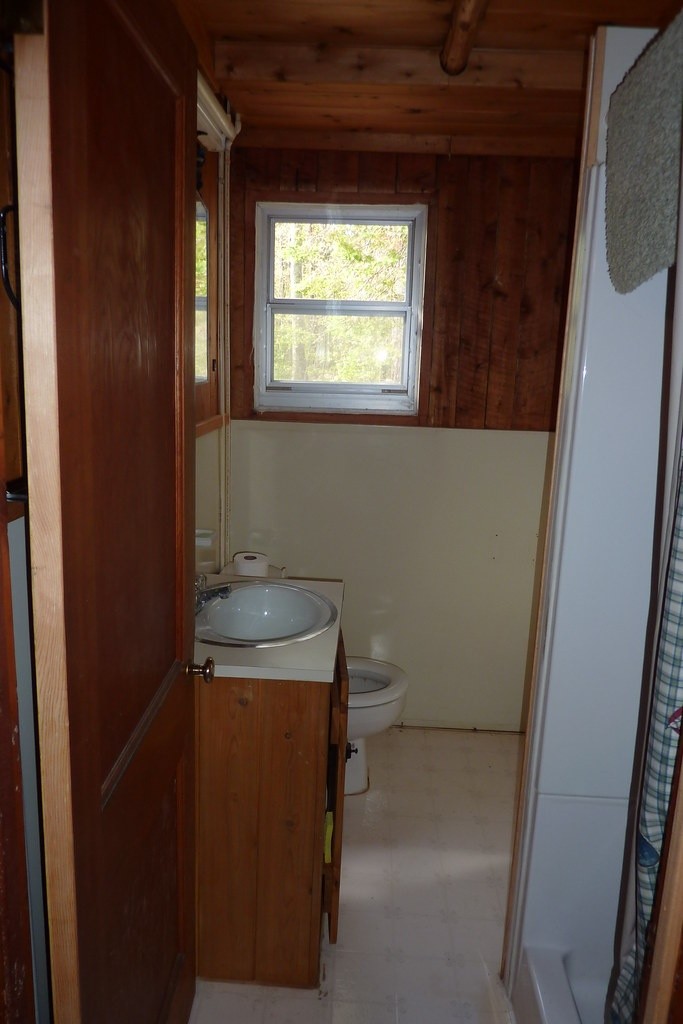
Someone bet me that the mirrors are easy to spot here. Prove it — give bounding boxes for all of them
[196,192,209,384]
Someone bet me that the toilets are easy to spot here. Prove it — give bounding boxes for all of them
[218,561,409,797]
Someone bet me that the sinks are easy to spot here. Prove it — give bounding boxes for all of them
[195,580,338,649]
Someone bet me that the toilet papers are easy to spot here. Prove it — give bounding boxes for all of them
[233,551,270,579]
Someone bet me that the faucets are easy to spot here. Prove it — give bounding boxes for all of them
[196,574,232,609]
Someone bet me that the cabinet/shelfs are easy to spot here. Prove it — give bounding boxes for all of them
[193,624,359,988]
